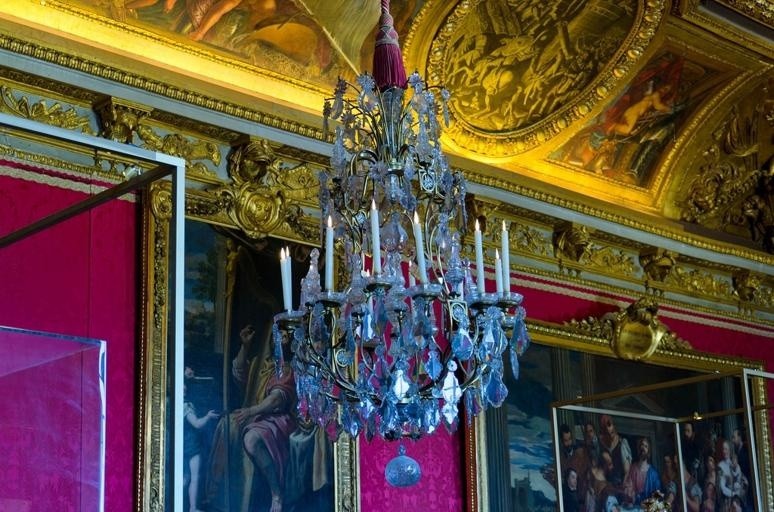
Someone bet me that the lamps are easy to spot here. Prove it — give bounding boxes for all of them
[272,68,530,487]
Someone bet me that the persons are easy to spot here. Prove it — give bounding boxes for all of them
[183,318,334,512]
[126,1,686,174]
[559,415,753,512]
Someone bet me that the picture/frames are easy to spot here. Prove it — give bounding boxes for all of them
[135,179,361,512]
[464,296,774,512]
[401,0,674,164]
